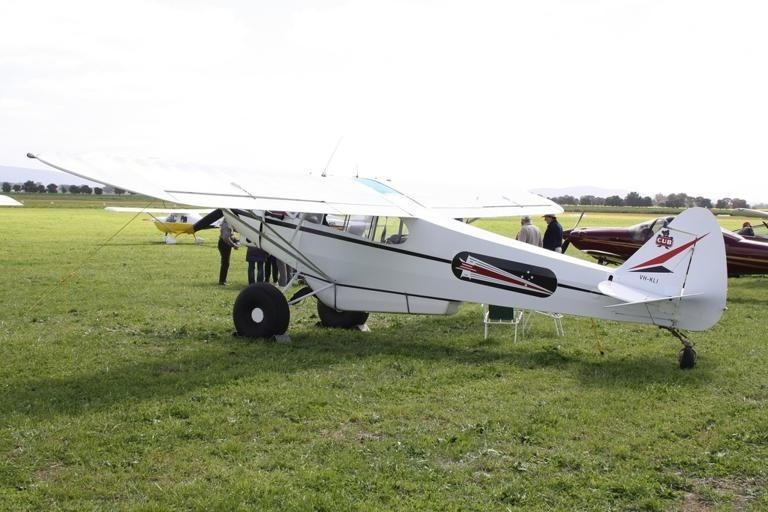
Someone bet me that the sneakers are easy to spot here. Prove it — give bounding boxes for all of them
[219,282,230,286]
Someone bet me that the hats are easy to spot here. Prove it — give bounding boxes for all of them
[543,214,556,217]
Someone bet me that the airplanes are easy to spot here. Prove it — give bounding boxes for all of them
[104,206,223,245]
[25,150,729,367]
[560,209,767,278]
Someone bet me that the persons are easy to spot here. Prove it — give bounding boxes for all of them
[737,221,755,237]
[217,215,240,287]
[542,212,563,255]
[245,235,303,287]
[515,215,543,250]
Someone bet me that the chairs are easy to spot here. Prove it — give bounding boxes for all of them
[480,301,524,344]
[524,310,565,337]
[641,228,654,239]
